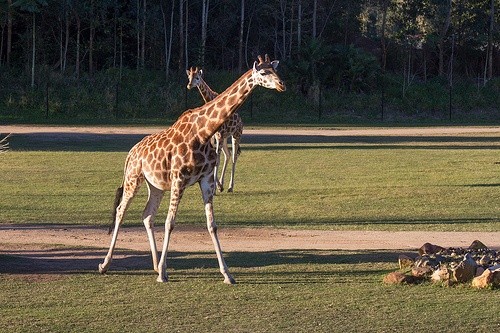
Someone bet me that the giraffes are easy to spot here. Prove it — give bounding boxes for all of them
[183,64,244,192]
[97,53,287,283]
